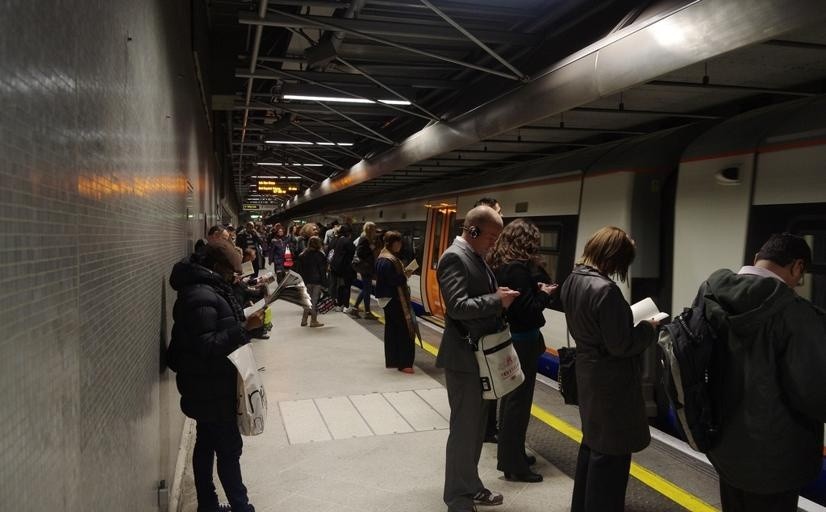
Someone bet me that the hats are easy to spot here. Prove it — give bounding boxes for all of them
[208,239,242,273]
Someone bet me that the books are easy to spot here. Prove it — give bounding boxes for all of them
[630,296,670,328]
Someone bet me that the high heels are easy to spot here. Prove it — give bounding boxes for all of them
[527,456,536,465]
[504,471,543,482]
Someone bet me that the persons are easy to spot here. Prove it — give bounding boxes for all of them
[435,205,520,512]
[487,218,559,483]
[560,226,658,512]
[691,231,826,512]
[195,218,417,374]
[167,238,268,512]
[474,197,504,443]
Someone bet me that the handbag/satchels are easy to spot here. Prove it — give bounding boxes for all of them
[227,343,266,436]
[657,280,717,452]
[557,347,576,404]
[478,323,525,399]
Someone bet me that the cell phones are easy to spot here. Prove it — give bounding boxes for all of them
[546,283,558,288]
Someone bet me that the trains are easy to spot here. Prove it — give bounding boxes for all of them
[268,118,826,505]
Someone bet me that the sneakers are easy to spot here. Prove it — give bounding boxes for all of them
[301,316,307,326]
[472,488,503,504]
[310,319,324,327]
[335,305,378,319]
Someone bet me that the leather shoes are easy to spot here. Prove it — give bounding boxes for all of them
[399,367,415,373]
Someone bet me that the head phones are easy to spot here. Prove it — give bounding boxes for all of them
[458,225,480,239]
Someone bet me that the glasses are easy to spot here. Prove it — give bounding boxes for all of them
[797,263,804,287]
